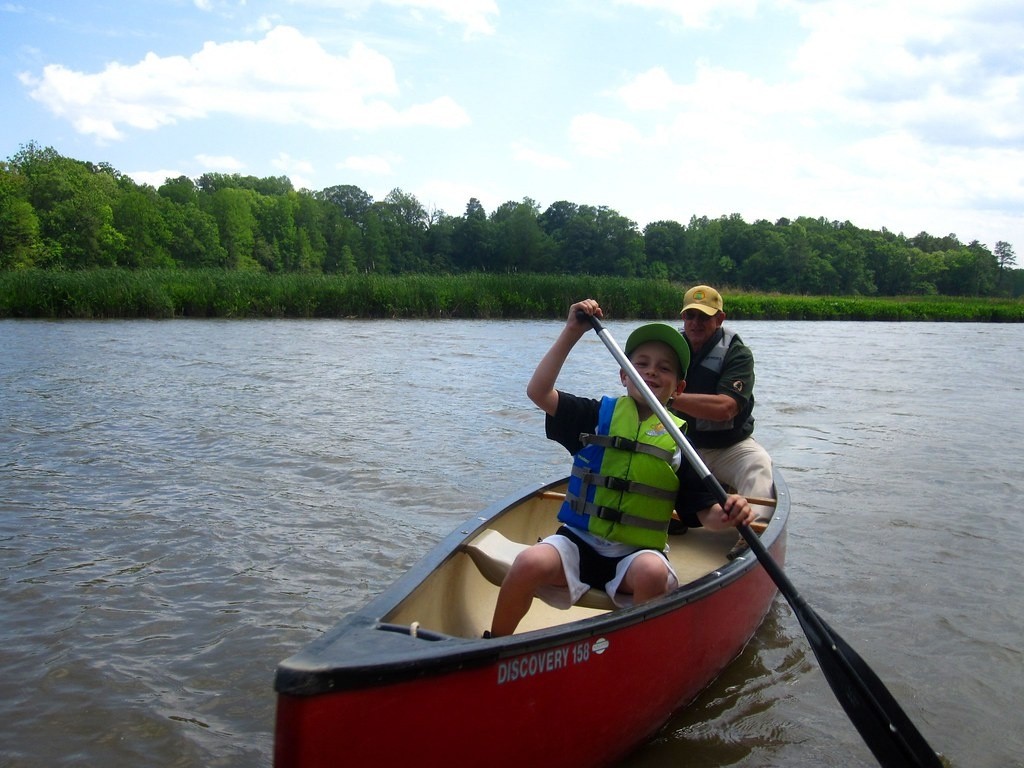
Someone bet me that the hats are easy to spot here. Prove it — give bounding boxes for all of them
[680,284,724,316]
[625,323,691,381]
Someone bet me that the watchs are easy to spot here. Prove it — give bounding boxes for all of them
[667,392,674,409]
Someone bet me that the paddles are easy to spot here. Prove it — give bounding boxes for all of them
[574,306,944,768]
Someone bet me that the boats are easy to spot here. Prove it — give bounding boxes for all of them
[274,443,793,768]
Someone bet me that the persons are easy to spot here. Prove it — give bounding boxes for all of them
[480,297,754,643]
[663,284,772,560]
